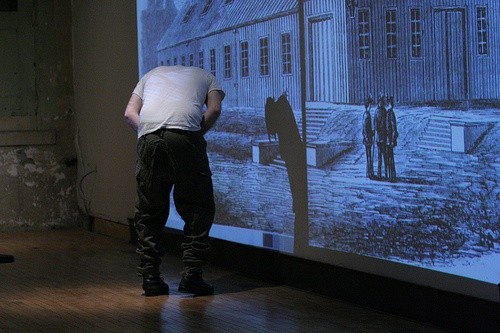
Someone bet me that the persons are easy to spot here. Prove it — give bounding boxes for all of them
[123,64,226,298]
[361,93,399,183]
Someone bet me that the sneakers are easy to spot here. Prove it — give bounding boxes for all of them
[178,277,214,297]
[142,277,169,295]
